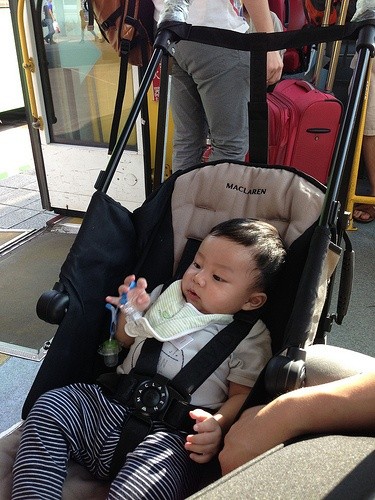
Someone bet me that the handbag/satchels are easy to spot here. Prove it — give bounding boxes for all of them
[42,19,47,27]
[53,21,61,33]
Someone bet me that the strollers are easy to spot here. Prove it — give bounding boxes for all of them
[22,0,375,438]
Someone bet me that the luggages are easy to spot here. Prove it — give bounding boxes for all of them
[245,78,344,186]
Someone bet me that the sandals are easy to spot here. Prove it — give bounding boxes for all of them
[352,189,375,224]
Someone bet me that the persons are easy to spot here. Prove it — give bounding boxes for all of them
[343,0,375,224]
[42,0,57,44]
[151,0,283,176]
[183,344,375,500]
[12,216,288,500]
[77,0,99,43]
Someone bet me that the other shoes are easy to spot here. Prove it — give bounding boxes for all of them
[44,36,49,43]
[49,40,56,44]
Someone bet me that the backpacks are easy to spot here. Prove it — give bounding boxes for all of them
[84,0,155,67]
